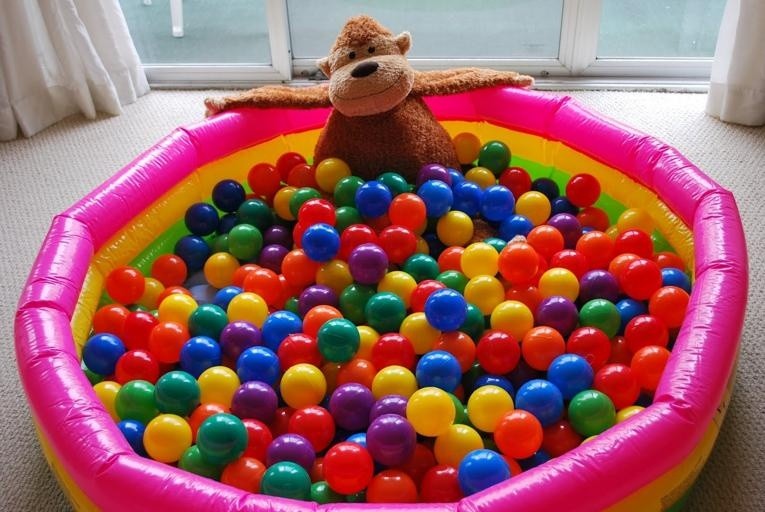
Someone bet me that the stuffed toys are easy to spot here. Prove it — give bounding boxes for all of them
[203,15,534,182]
